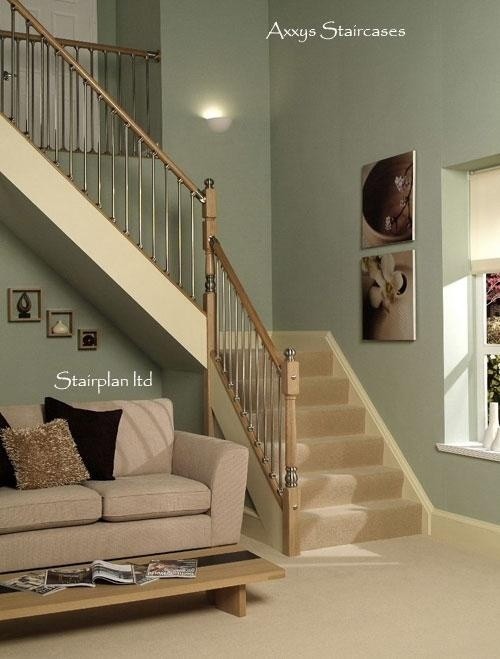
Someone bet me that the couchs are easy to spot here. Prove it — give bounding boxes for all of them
[1,397,250,572]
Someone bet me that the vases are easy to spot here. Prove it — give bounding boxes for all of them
[490,427,500,452]
[396,271,407,297]
[482,403,499,449]
[360,151,413,240]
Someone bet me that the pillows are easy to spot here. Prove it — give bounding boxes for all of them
[0,411,17,489]
[1,418,90,491]
[43,397,123,480]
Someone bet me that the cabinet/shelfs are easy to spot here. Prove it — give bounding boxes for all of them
[8,288,100,350]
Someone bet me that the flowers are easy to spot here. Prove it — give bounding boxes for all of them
[384,162,413,233]
[360,253,401,313]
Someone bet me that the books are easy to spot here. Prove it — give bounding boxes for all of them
[1,557,198,597]
